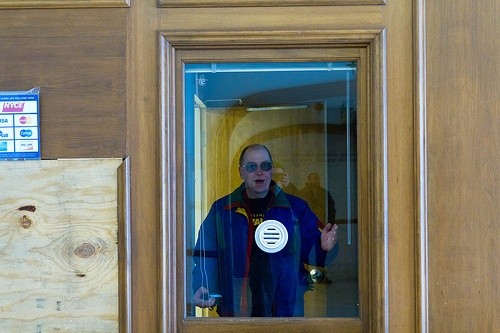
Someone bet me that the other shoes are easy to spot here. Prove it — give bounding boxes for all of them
[316,272,333,285]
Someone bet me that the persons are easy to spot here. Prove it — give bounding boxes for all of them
[192,144,337,318]
[270,166,335,285]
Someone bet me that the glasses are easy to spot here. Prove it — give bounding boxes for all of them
[243,160,272,173]
